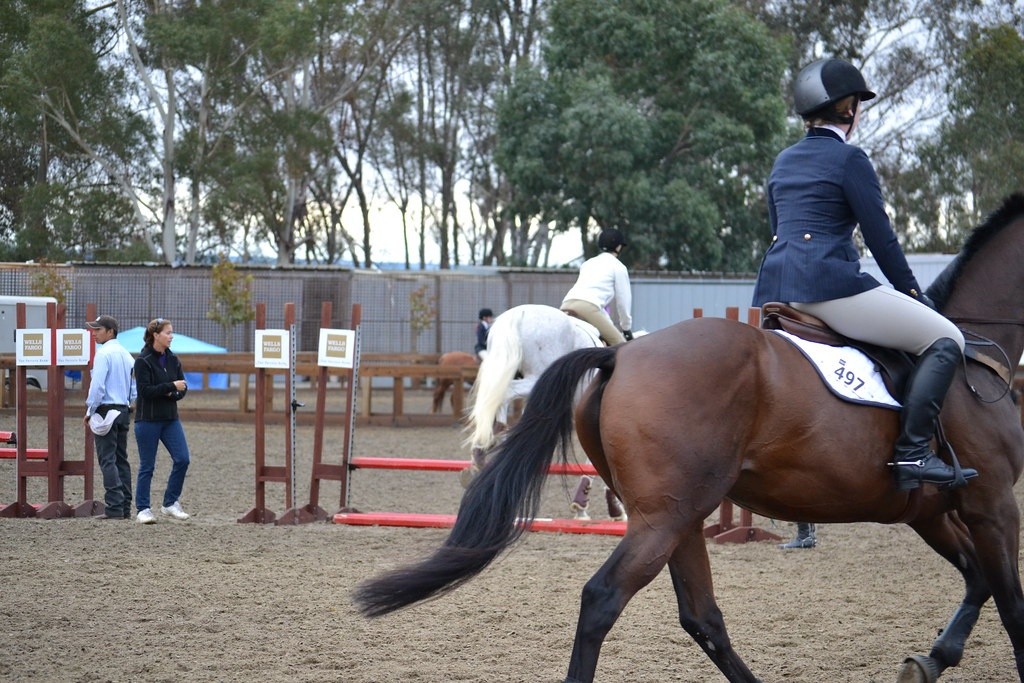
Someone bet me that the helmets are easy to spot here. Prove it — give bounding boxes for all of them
[599,228,628,250]
[479,308,492,319]
[795,59,876,115]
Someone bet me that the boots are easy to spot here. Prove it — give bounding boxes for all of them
[778,521,818,547]
[892,337,978,489]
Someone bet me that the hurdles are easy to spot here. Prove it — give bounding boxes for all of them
[236,300,784,545]
[0,301,108,520]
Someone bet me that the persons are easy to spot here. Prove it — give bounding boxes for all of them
[753,60,978,490]
[778,521,816,548]
[474,308,493,359]
[84,314,137,522]
[559,227,633,344]
[131,318,190,524]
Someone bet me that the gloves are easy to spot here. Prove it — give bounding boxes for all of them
[921,294,935,311]
[624,329,632,340]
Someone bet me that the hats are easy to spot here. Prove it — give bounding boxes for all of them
[86,316,119,333]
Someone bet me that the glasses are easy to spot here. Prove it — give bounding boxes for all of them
[153,318,163,330]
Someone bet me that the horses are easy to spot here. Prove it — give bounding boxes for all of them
[349,188,1024,683]
[458,304,651,522]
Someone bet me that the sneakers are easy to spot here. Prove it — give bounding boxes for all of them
[161,501,190,520]
[137,507,156,523]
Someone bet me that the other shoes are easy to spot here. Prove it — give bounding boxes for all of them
[96,513,126,520]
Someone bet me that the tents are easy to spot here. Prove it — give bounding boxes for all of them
[94,325,232,394]
